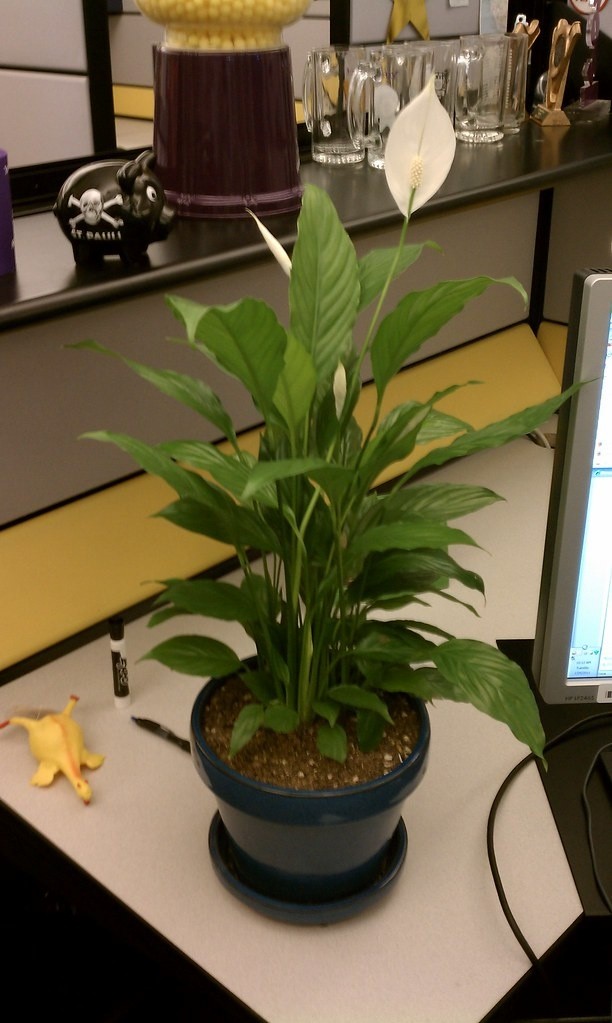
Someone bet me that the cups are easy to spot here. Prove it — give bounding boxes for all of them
[302,45,367,164]
[451,35,513,144]
[346,44,437,170]
[484,33,528,131]
[402,39,461,138]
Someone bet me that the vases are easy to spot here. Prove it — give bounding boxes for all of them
[193,654,431,924]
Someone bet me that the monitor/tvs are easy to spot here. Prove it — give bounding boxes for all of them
[531,267,612,704]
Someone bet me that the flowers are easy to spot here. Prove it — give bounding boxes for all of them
[58,74,557,778]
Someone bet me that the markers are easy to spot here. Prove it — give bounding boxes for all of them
[107,615,131,707]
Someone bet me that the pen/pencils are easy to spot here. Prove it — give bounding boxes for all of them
[127,716,193,753]
